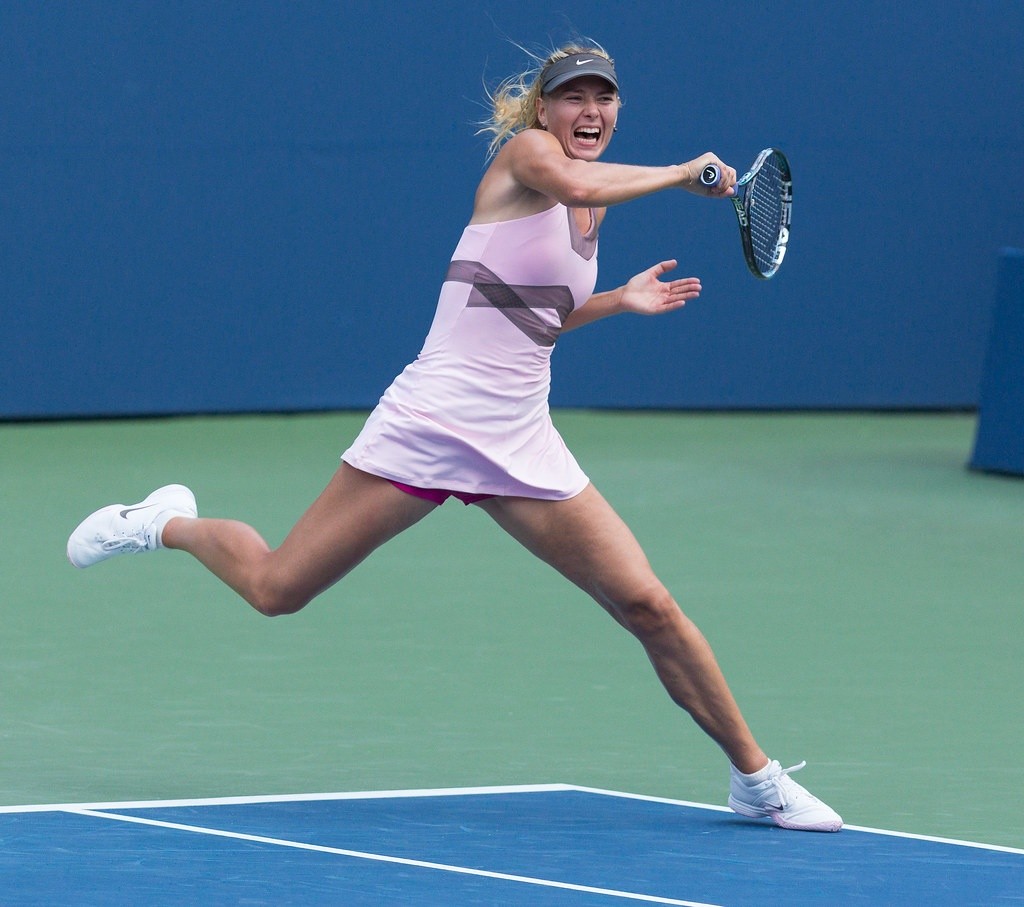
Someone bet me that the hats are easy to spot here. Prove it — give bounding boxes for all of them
[540,53,619,97]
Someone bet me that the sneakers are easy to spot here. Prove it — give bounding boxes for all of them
[727,760,844,832]
[67,484,199,569]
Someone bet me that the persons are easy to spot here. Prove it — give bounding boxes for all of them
[66,13,843,833]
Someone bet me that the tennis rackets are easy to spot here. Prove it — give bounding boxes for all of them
[698,146,794,282]
